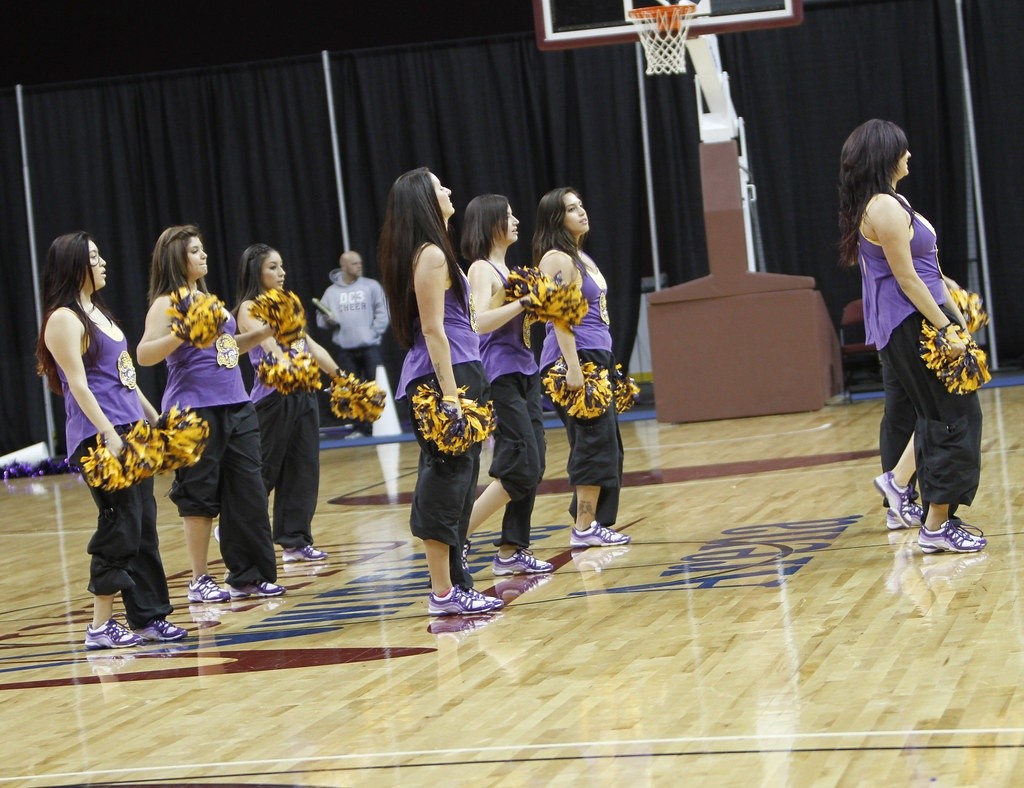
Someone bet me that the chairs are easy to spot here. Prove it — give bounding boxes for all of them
[840,299,880,401]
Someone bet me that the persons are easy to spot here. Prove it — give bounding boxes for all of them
[460,195,567,575]
[381,167,505,616]
[136,226,286,603]
[36,232,188,648]
[842,117,987,554]
[315,251,390,440]
[538,188,630,547]
[214,244,348,562]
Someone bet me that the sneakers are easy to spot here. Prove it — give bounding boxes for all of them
[873,471,915,529]
[569,520,632,549]
[921,551,989,585]
[427,609,497,643]
[86,647,139,676]
[917,519,988,554]
[282,544,329,564]
[886,500,923,530]
[492,547,554,576]
[494,571,553,600]
[570,544,631,573]
[462,538,472,575]
[228,581,286,601]
[188,603,233,629]
[344,431,372,441]
[133,618,188,642]
[428,584,495,617]
[463,587,505,610]
[84,617,144,651]
[187,574,232,604]
